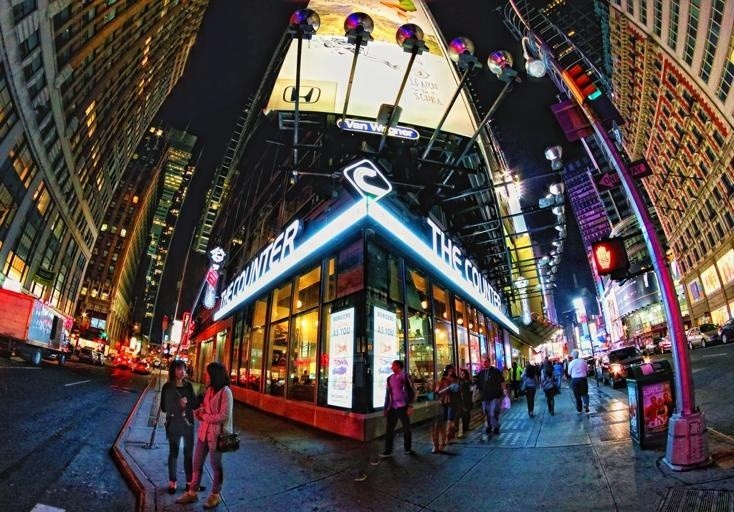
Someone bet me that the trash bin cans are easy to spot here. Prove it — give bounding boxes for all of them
[626,359,675,450]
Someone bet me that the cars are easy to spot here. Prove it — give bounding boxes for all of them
[718,319,733,344]
[582,323,672,390]
[686,324,722,349]
[69,342,174,377]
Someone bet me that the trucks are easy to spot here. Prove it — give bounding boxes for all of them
[0,288,78,367]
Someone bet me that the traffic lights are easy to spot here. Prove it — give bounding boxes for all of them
[590,236,631,276]
[560,61,597,106]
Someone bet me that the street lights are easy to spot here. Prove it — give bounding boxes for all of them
[514,27,718,475]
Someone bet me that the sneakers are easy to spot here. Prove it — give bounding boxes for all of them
[184,484,206,492]
[204,494,221,508]
[378,451,395,457]
[169,485,176,495]
[176,492,198,503]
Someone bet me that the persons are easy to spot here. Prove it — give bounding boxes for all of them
[301,370,311,385]
[653,333,662,354]
[175,362,233,508]
[160,360,209,494]
[377,350,592,459]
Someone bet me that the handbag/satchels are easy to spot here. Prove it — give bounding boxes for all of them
[216,433,239,452]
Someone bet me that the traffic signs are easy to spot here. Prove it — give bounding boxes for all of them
[590,157,653,192]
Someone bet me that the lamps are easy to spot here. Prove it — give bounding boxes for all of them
[287,10,567,300]
